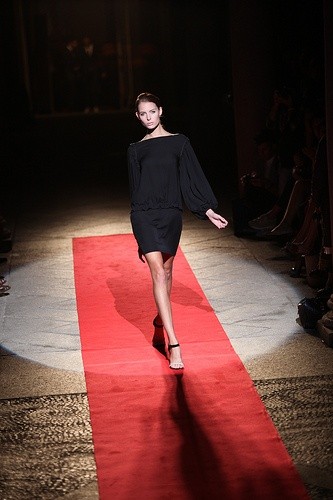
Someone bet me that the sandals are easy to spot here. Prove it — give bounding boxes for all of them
[168,344,184,369]
[152,316,164,345]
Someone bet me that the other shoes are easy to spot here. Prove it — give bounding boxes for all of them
[0,285,9,292]
[289,258,304,277]
[0,279,7,285]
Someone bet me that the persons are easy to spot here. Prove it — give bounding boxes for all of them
[239,88,333,347]
[126,92,228,369]
